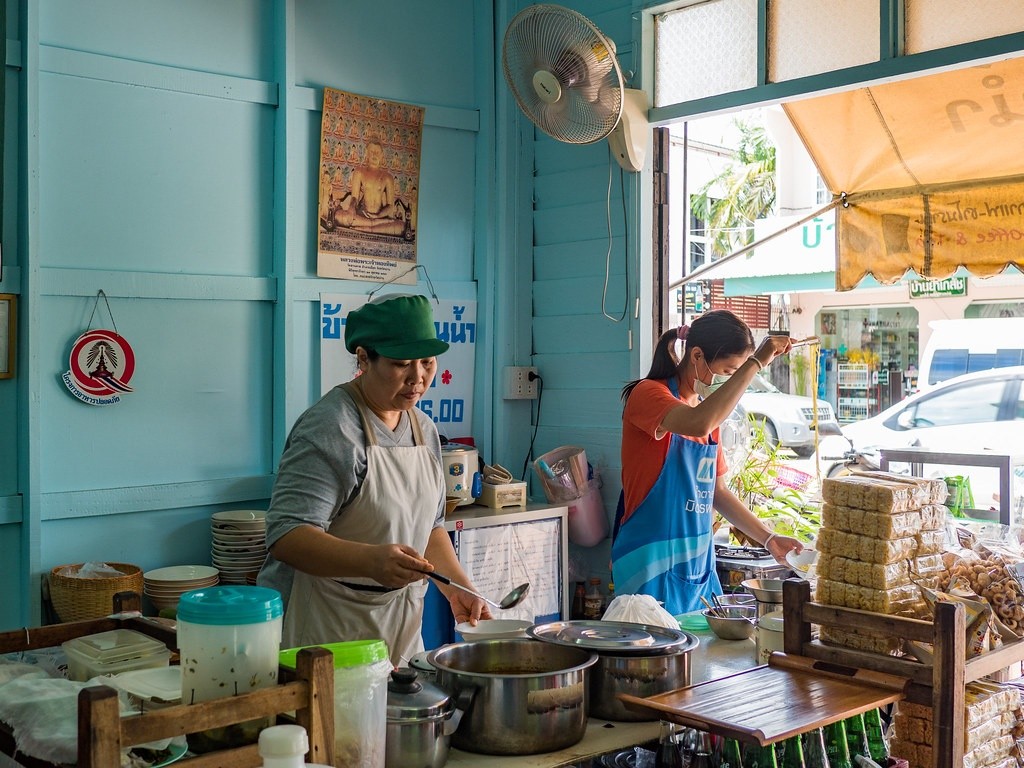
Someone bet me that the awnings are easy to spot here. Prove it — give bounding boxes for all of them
[669,58,1024,290]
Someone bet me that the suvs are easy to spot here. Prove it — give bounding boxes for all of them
[731,374,840,462]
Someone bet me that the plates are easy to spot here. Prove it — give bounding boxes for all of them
[152,742,188,768]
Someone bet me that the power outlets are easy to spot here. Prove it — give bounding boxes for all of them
[503,366,538,399]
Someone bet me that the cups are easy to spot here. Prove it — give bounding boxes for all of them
[757,601,784,625]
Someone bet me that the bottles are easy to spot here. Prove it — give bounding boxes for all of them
[257,724,310,768]
[571,579,615,620]
[653,706,890,768]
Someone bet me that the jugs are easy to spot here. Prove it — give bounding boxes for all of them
[175,584,285,754]
[280,637,389,768]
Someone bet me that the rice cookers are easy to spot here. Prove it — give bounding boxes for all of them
[438,434,483,507]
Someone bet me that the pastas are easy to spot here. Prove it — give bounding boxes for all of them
[801,563,809,572]
[808,341,821,488]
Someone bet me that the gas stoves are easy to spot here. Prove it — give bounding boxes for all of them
[714,544,796,595]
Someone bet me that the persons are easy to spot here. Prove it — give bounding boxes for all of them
[323,90,420,235]
[612,310,805,614]
[258,293,495,669]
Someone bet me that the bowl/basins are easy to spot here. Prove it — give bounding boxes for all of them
[701,606,757,641]
[445,496,462,518]
[712,595,756,606]
[143,508,269,613]
[455,619,534,642]
[785,549,819,579]
[61,630,181,712]
[741,579,784,602]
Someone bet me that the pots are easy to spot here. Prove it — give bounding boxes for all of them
[587,630,700,722]
[386,668,452,768]
[428,638,600,756]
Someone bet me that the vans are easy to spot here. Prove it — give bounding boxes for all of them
[905,318,1024,422]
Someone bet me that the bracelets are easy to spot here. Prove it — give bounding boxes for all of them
[765,533,776,551]
[748,356,762,372]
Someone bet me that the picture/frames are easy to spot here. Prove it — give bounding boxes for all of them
[820,313,837,335]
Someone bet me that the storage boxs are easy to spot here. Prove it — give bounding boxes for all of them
[474,480,527,509]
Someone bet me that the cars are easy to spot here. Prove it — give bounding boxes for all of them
[810,364,1024,518]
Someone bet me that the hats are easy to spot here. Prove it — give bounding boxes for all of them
[344,293,450,359]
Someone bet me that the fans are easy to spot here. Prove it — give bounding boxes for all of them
[502,4,649,173]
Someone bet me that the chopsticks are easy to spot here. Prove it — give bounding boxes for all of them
[792,335,822,349]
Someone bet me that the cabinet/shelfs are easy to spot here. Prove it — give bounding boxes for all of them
[818,349,878,422]
[868,331,919,370]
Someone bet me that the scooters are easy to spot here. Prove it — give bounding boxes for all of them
[814,422,922,476]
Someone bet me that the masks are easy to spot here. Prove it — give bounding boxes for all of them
[694,361,732,399]
